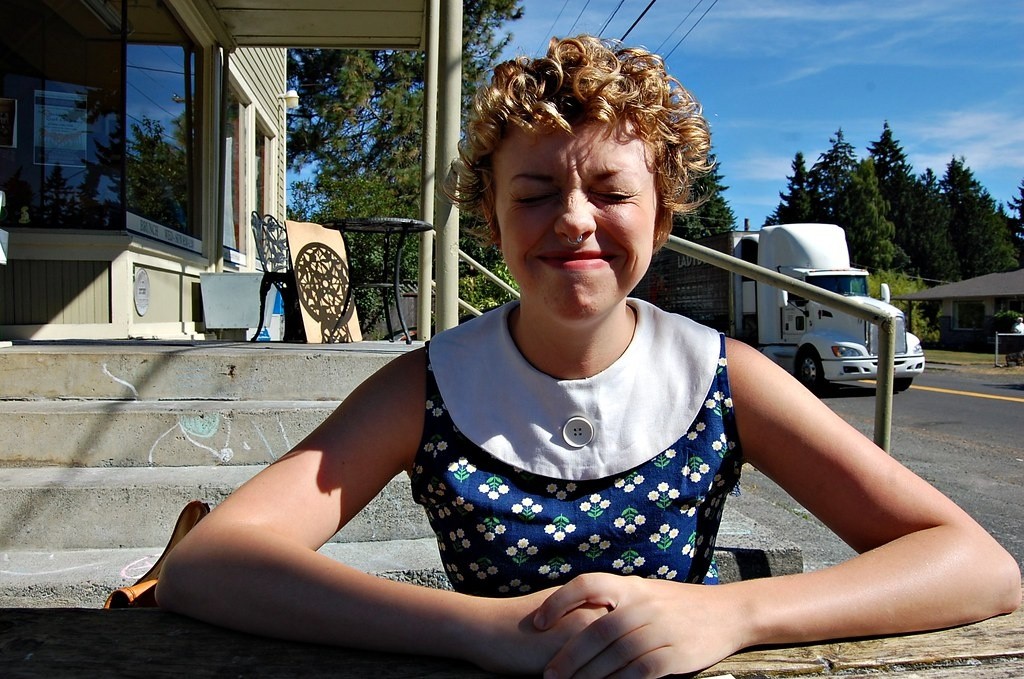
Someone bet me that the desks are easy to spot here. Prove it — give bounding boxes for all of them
[1,587,1023,678]
[200,272,278,339]
[322,217,434,345]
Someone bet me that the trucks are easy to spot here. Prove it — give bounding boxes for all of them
[626,223,927,398]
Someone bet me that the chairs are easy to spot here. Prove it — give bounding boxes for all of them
[251,211,307,344]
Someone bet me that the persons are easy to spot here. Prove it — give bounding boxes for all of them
[155,36,1024,679]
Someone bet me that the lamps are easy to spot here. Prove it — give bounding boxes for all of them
[278,90,299,108]
